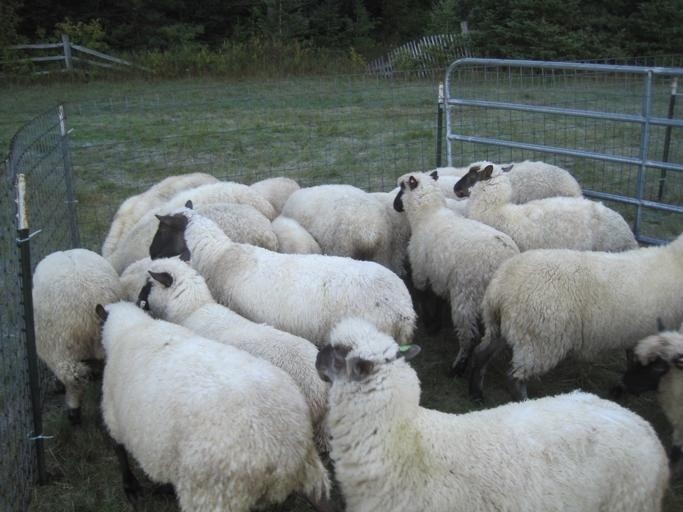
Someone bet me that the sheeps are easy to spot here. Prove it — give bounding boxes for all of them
[31,158,682,512]
